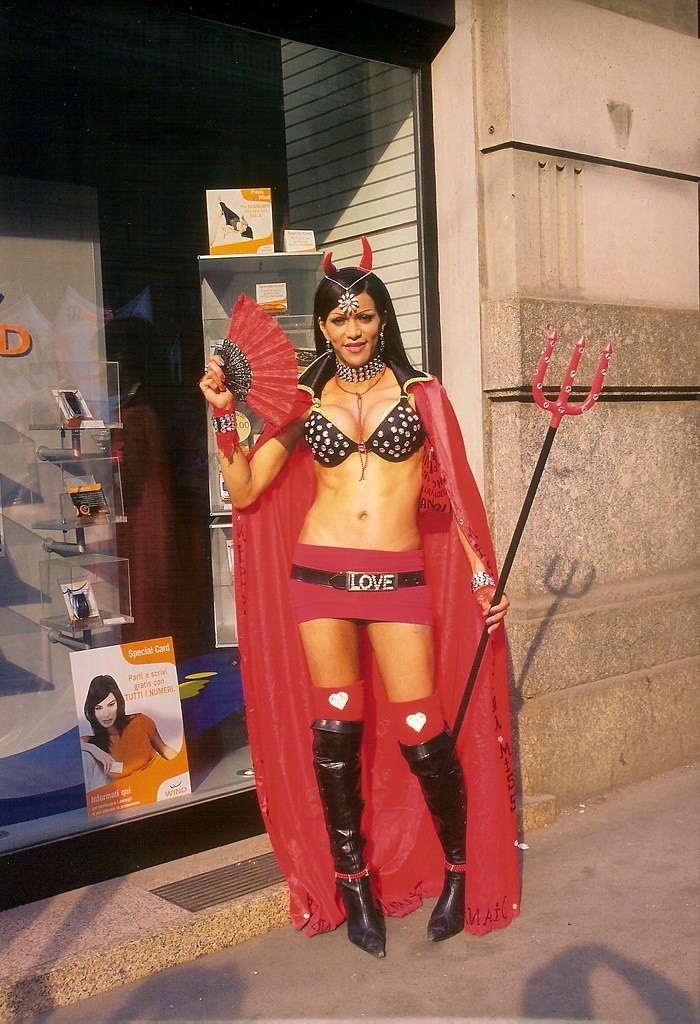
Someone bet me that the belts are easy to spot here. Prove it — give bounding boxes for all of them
[290,563,426,591]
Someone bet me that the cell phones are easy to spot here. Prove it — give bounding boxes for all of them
[59,391,83,419]
[67,589,89,619]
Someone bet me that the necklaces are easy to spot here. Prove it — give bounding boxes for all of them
[332,359,387,480]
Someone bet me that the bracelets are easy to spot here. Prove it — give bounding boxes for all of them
[212,412,237,433]
[472,572,495,591]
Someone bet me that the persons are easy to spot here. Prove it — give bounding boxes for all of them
[57,313,205,673]
[199,235,520,959]
[80,675,178,783]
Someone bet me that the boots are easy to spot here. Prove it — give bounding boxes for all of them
[309,718,389,957]
[398,723,467,943]
